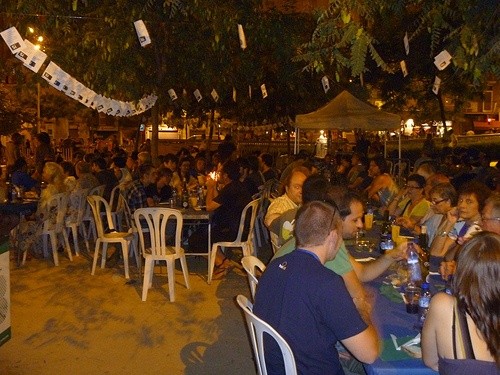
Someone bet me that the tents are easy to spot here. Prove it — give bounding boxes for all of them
[294,90,401,184]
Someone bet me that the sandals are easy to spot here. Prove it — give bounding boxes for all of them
[213,257,230,279]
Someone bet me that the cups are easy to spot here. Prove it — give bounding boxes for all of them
[405,286,422,313]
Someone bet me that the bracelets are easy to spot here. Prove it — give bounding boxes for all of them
[385,248,408,260]
[393,197,399,201]
[438,231,448,237]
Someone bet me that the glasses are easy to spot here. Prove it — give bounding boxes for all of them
[407,186,419,190]
[316,198,337,235]
[481,216,500,222]
[431,199,445,205]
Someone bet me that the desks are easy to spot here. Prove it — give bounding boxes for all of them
[335,204,453,375]
[0,197,40,267]
[131,203,214,285]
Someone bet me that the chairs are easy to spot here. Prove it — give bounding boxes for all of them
[236,256,298,375]
[21,185,140,279]
[133,208,189,301]
[206,179,273,283]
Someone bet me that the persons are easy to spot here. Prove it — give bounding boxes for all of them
[421,230,499,374]
[0,125,500,292]
[251,200,380,375]
[268,188,410,360]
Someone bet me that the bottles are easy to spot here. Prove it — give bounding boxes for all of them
[418,283,432,327]
[405,242,422,281]
[173,182,207,211]
[380,210,392,254]
